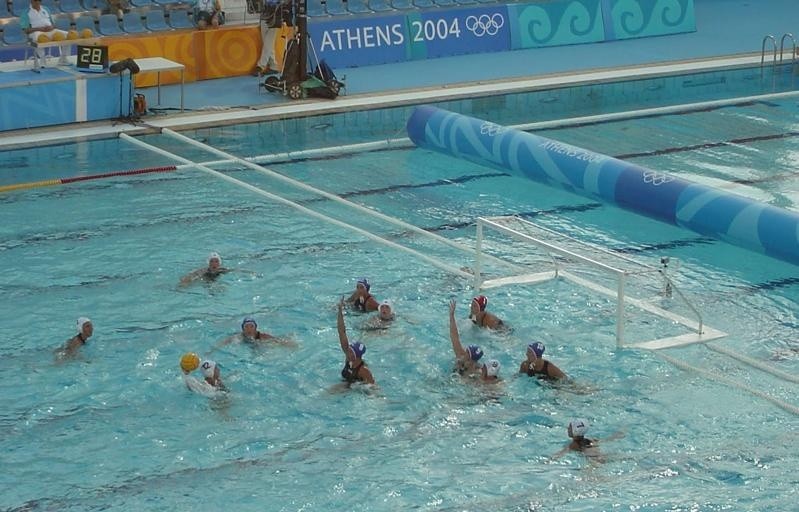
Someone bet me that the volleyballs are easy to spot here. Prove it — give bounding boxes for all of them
[67,29,81,40]
[52,30,65,40]
[82,29,93,38]
[38,32,49,42]
[181,352,199,371]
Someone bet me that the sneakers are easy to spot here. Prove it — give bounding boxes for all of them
[254,66,264,77]
[264,69,279,75]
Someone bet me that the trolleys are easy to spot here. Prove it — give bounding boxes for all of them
[259,9,347,100]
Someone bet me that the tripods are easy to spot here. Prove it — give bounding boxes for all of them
[279,31,325,81]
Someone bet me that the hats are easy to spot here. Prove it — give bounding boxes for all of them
[199,360,217,379]
[77,316,91,334]
[207,252,222,267]
[377,299,393,316]
[465,343,483,362]
[241,317,257,332]
[356,278,371,294]
[483,359,501,378]
[527,341,545,361]
[348,341,366,361]
[570,418,590,439]
[473,295,488,313]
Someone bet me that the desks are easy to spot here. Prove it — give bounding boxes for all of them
[109,57,185,113]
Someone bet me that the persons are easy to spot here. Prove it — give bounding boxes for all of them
[469,296,506,332]
[334,276,379,312]
[449,297,485,388]
[461,358,502,387]
[21,1,74,46]
[520,342,570,382]
[51,316,97,369]
[171,1,223,31]
[252,1,282,76]
[324,292,374,395]
[182,361,231,401]
[216,318,301,346]
[168,253,261,287]
[540,418,626,484]
[353,297,398,339]
